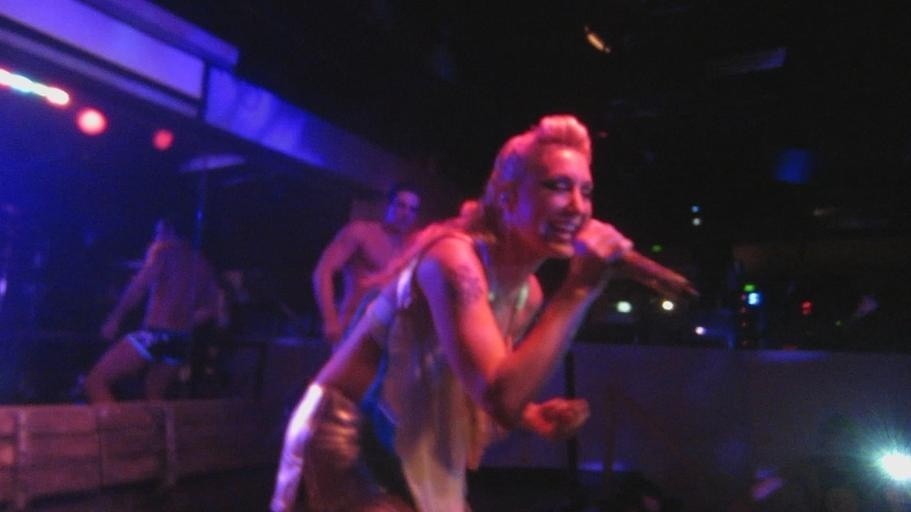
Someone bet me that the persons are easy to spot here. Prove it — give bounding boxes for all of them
[86,211,232,500]
[268,112,636,512]
[312,178,425,355]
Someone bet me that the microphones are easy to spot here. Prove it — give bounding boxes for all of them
[615,251,701,303]
